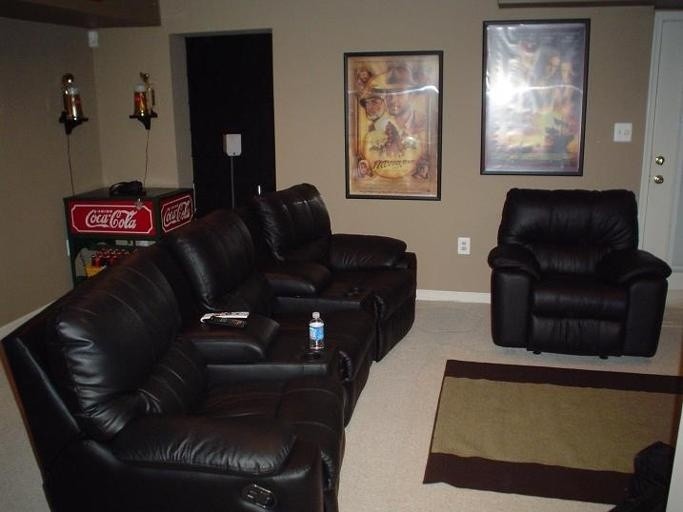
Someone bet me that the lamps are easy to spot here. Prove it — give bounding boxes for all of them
[58,73,87,136]
[127,72,158,130]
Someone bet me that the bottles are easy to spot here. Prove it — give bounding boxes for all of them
[308,312,323,354]
[91,247,128,268]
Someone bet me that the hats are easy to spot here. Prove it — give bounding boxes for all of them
[373,65,425,95]
[359,77,387,105]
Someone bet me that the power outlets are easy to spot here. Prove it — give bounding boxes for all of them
[457,236,471,256]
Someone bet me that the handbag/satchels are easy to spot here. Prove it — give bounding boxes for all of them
[608,440,674,511]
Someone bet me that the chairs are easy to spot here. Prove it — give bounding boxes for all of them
[486,186,673,359]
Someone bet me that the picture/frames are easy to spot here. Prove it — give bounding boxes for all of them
[479,17,590,178]
[342,50,443,202]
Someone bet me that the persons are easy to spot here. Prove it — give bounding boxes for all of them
[370,66,423,135]
[358,83,386,124]
[356,157,372,178]
[411,158,432,181]
[354,67,369,90]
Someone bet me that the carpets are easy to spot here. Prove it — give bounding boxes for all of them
[422,357,682,506]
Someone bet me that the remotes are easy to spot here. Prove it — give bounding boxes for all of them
[205,316,247,329]
[200,311,250,323]
